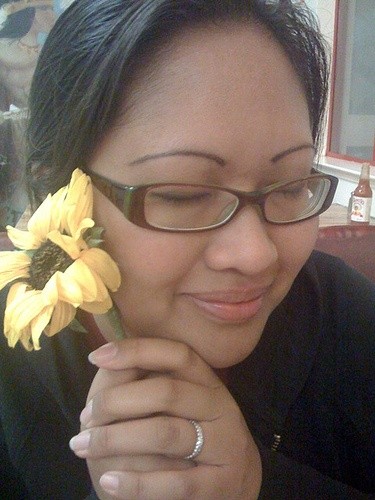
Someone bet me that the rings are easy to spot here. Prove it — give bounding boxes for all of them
[183,419,204,460]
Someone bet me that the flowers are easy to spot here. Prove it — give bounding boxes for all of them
[0,169,126,352]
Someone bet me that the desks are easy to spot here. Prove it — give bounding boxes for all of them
[316,205,374,286]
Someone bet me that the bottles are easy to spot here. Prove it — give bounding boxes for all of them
[351,163,373,225]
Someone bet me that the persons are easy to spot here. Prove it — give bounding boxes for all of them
[0,0,375,500]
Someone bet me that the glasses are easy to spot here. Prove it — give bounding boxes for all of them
[82,166,339,232]
